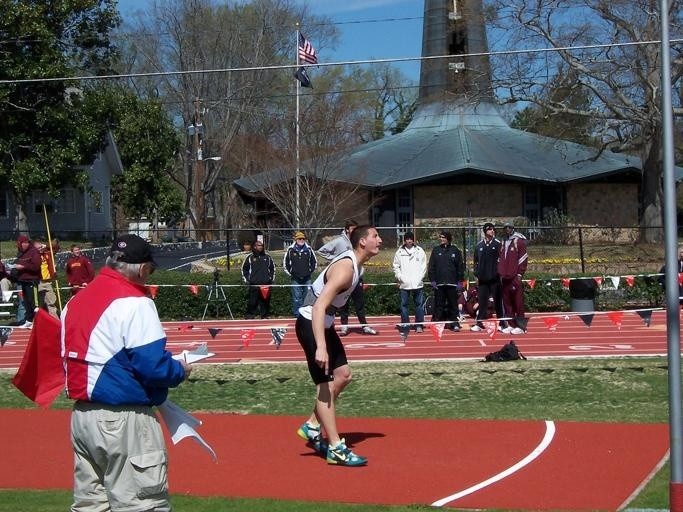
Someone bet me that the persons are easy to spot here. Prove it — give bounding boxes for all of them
[677,246,683,308]
[57,235,193,511]
[0,234,94,330]
[316,218,378,337]
[428,229,466,331]
[469,224,508,334]
[294,225,383,466]
[658,256,667,308]
[282,231,318,320]
[495,219,530,335]
[391,231,429,337]
[240,240,277,320]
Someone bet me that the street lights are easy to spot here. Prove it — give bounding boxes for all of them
[197,157,220,242]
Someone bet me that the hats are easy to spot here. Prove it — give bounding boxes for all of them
[296,232,305,240]
[404,232,414,242]
[503,220,514,228]
[483,223,493,234]
[110,235,153,264]
[18,235,28,242]
[441,231,451,242]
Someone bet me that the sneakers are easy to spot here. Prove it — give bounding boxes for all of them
[19,320,33,329]
[297,421,327,458]
[497,326,527,334]
[453,324,485,332]
[415,327,423,333]
[327,438,367,466]
[339,327,376,335]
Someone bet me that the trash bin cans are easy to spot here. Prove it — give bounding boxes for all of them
[569,280,594,312]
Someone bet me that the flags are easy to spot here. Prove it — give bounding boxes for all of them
[10,306,65,409]
[157,399,218,462]
[297,31,320,69]
[294,64,315,89]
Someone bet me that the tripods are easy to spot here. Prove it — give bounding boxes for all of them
[202,281,234,320]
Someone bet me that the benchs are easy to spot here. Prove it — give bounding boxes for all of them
[0,289,22,316]
